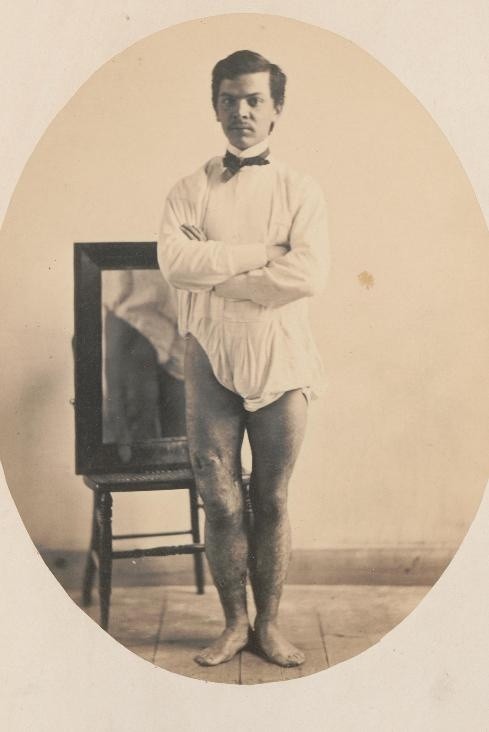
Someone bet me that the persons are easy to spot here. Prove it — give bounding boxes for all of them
[156,49,329,667]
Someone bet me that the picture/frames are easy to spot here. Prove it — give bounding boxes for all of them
[73,242,199,473]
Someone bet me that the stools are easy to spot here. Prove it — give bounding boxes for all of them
[81,470,253,633]
